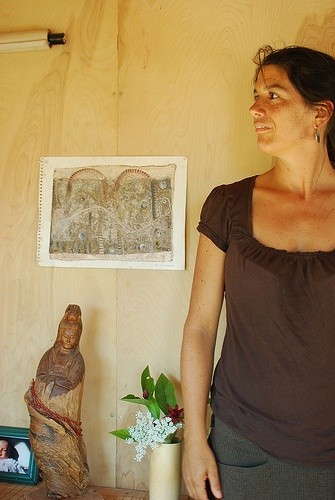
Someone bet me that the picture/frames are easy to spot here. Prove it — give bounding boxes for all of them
[0,426,40,486]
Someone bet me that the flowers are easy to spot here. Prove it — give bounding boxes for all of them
[107,365,211,463]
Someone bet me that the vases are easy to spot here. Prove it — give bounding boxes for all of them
[148,442,182,500]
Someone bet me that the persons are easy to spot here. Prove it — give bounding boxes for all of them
[25,304,92,499]
[180,46,335,500]
[0,440,25,474]
